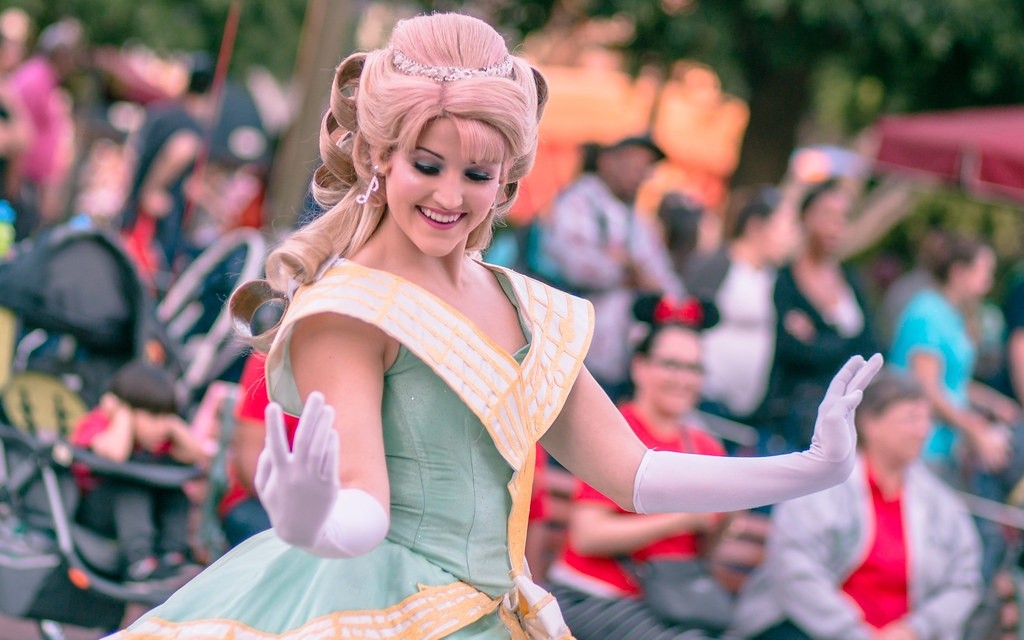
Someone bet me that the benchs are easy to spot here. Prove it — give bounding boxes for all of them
[178,382,1024,640]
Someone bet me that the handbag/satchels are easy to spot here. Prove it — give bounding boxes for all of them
[637,558,737,631]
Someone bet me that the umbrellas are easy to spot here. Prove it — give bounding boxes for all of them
[857,106,1024,206]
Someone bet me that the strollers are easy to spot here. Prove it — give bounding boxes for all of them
[0,215,215,626]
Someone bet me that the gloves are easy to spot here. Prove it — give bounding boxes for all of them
[632,352,885,514]
[253,391,390,559]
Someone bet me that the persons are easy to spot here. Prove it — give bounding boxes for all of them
[463,136,1023,640]
[0,5,330,640]
[97,6,885,640]
